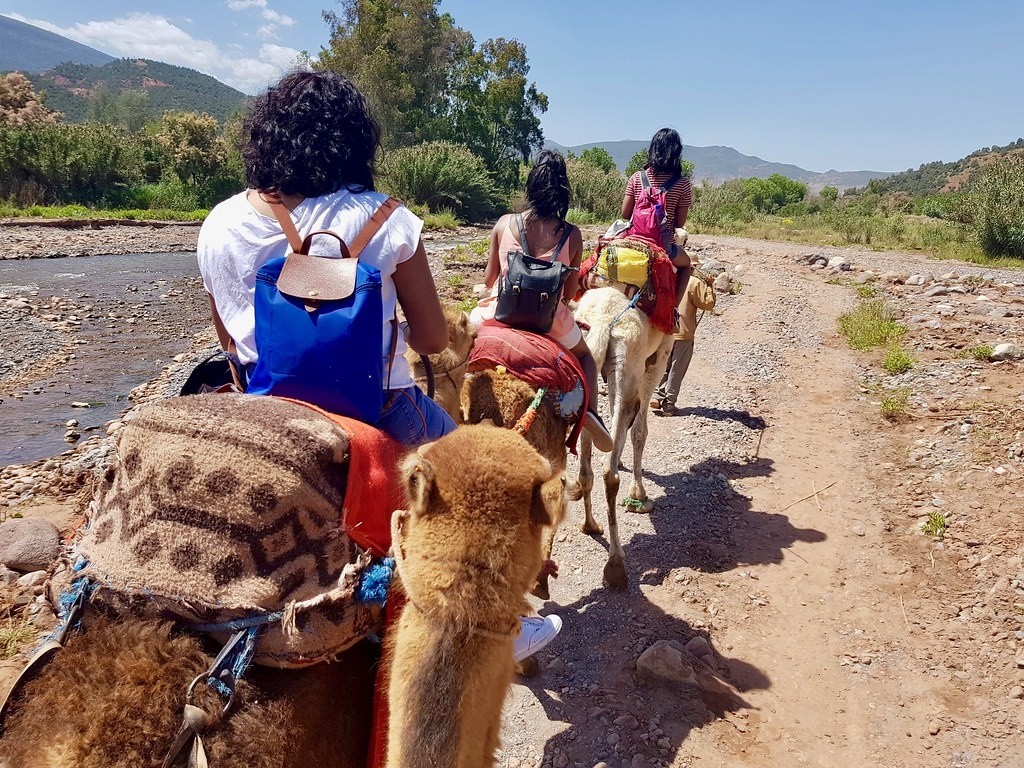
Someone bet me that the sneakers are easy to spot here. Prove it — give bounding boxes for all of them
[650,398,661,409]
[515,613,562,662]
[674,308,680,334]
[583,407,614,453]
[663,408,679,416]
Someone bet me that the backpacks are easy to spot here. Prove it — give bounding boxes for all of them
[621,166,683,251]
[244,179,404,423]
[494,212,575,333]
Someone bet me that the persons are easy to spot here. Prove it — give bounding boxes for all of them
[648,251,717,413]
[609,126,690,334]
[469,150,614,453]
[195,70,564,662]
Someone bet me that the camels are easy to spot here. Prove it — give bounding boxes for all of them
[380,417,568,768]
[0,284,678,767]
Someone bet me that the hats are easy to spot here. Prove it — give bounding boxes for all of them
[685,250,702,265]
